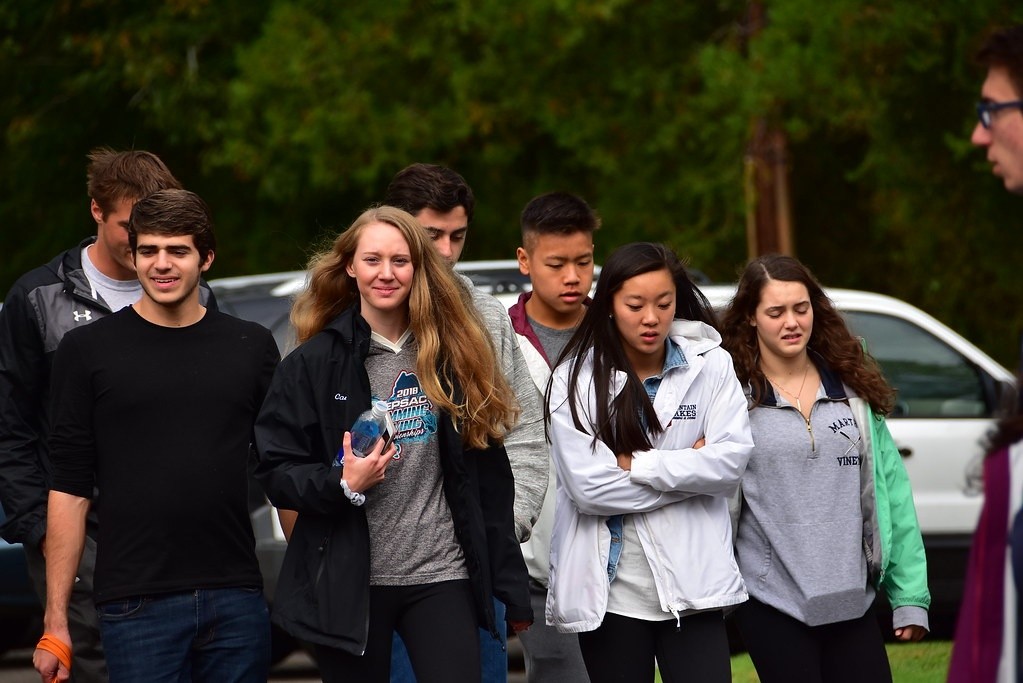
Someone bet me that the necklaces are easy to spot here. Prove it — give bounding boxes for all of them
[758,356,810,413]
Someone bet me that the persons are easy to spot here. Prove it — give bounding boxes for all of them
[543,240,752,683]
[717,252,932,682]
[934,10,1023,683]
[500,186,596,683]
[0,147,540,683]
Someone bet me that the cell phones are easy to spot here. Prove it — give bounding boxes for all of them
[381,412,398,456]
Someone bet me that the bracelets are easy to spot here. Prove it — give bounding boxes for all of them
[341,477,367,506]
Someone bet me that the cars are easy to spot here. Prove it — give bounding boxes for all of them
[694,284,1023,606]
[205,260,605,364]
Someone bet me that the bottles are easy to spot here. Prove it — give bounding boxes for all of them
[331,401,388,467]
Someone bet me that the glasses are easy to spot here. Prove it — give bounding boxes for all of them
[976,100,1022,128]
